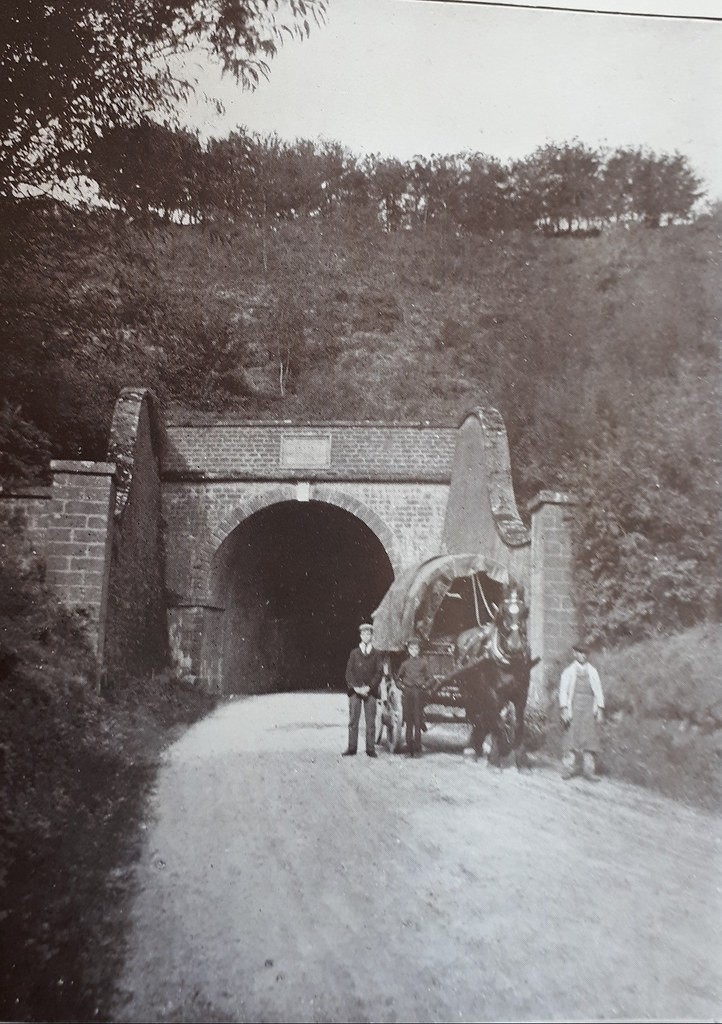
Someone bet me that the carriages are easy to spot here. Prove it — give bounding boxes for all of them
[370,552,541,772]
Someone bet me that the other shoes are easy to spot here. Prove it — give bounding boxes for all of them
[342,748,357,756]
[404,750,422,759]
[366,750,377,758]
[562,771,573,780]
[583,772,601,782]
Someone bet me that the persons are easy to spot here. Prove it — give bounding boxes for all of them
[341,623,383,758]
[558,641,605,783]
[394,637,435,759]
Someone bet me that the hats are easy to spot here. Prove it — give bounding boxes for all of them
[359,623,373,630]
[572,643,589,652]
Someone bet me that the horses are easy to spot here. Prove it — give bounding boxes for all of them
[455,583,532,775]
